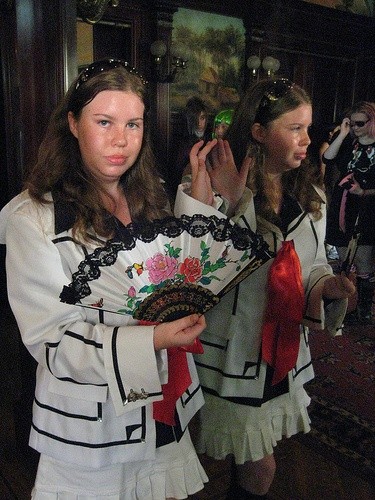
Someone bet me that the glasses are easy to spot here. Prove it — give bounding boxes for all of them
[255,78,296,122]
[74,58,151,91]
[350,118,370,127]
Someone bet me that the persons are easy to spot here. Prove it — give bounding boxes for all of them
[6,58,216,500]
[169,77,375,500]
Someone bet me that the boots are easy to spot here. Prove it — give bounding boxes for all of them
[355,275,372,321]
